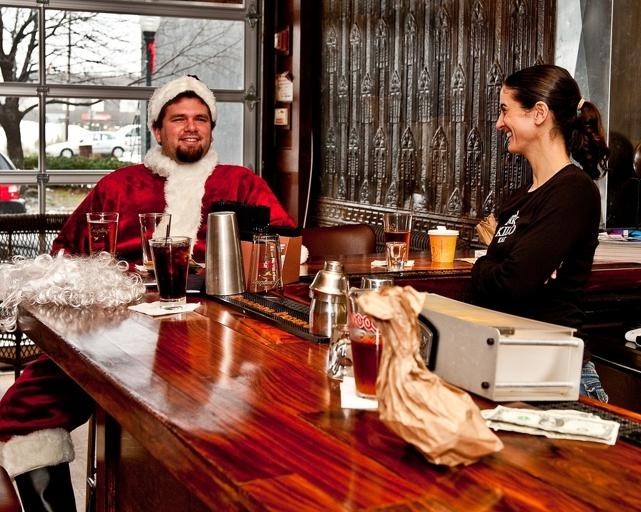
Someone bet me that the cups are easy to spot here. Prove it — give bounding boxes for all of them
[203,211,247,298]
[85,211,120,257]
[428,227,460,263]
[385,241,409,273]
[344,289,384,400]
[137,211,173,272]
[382,209,412,263]
[147,236,192,310]
[359,272,394,293]
[247,233,283,294]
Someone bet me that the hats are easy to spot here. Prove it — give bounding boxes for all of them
[148,74,217,131]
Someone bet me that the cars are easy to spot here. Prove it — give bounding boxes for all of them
[41,130,126,160]
[38,125,89,144]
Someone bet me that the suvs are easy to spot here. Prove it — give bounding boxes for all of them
[0,151,25,212]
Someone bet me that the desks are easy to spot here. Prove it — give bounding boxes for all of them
[16,243,641,512]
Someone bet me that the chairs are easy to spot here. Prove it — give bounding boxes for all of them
[298,224,377,254]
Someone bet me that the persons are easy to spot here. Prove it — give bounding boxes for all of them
[469,62,610,407]
[0,73,309,512]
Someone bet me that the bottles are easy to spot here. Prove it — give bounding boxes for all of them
[305,259,350,339]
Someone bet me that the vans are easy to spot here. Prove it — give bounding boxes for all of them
[117,124,140,149]
